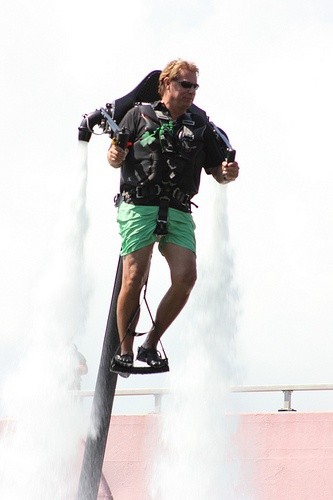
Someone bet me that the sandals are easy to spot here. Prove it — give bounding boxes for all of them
[139,346,164,366]
[114,354,133,366]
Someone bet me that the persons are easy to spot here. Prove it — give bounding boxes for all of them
[106,58,239,370]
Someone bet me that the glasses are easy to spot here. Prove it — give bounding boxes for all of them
[175,81,200,90]
